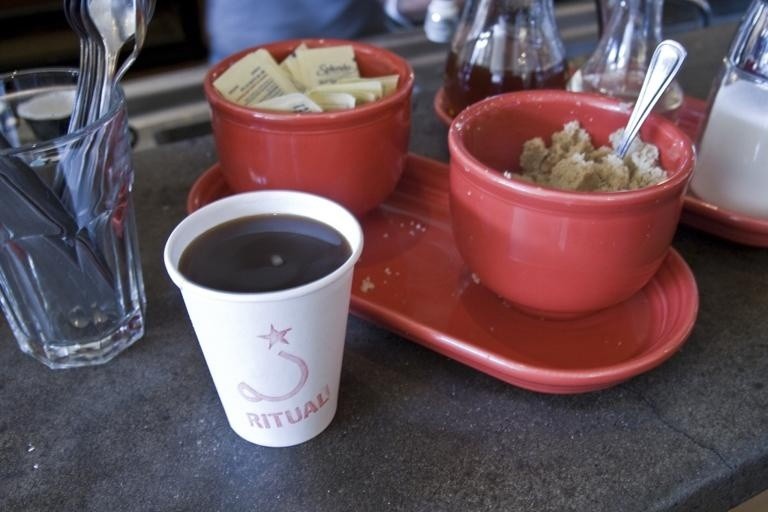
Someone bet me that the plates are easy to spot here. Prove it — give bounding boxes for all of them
[181,143,703,396]
[433,69,764,243]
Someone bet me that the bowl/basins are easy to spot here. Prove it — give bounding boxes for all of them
[200,34,417,217]
[448,89,697,320]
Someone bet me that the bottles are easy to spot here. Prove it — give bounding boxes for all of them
[571,0,690,148]
[450,1,569,117]
[691,1,768,220]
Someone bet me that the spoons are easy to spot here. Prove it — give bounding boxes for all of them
[54,0,158,326]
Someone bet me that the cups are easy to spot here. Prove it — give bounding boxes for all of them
[162,188,367,448]
[0,60,148,378]
